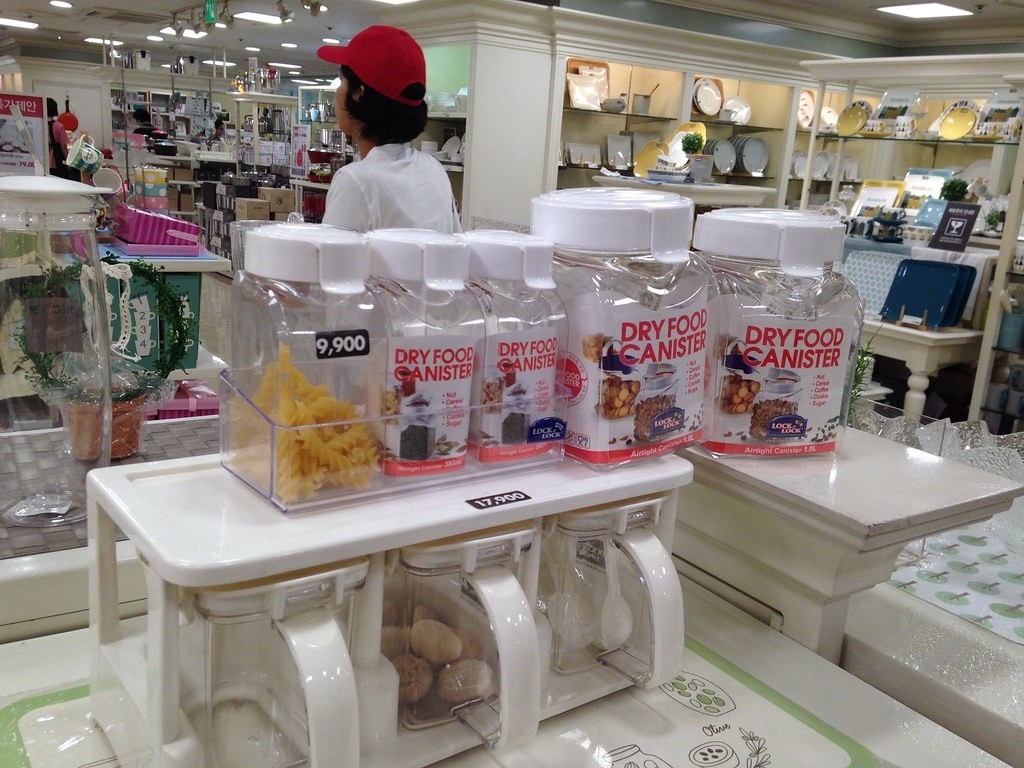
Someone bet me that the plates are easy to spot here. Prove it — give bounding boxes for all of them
[441,136,461,158]
[565,66,1003,222]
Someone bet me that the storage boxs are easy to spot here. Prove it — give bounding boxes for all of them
[153,164,174,181]
[221,238,233,270]
[146,402,159,422]
[134,182,167,196]
[212,210,224,236]
[256,139,273,154]
[210,236,223,257]
[179,194,193,212]
[272,153,290,166]
[258,186,295,214]
[234,176,253,186]
[202,181,217,210]
[205,207,214,250]
[215,184,232,210]
[168,199,179,211]
[172,167,193,181]
[160,377,220,419]
[242,151,254,165]
[221,363,572,518]
[221,174,235,184]
[223,212,236,238]
[227,184,251,213]
[135,195,168,209]
[113,201,202,257]
[168,184,179,199]
[234,197,270,221]
[270,140,290,154]
[151,209,169,215]
[133,167,167,184]
[270,212,289,221]
[258,154,272,166]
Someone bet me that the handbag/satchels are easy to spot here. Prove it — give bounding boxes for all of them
[53,144,65,163]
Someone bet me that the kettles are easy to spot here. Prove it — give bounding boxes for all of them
[244,115,254,132]
[271,109,285,133]
[259,117,270,133]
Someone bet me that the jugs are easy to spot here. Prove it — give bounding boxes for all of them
[229,212,385,500]
[692,208,859,462]
[381,520,542,751]
[364,228,487,479]
[530,187,722,472]
[177,555,371,768]
[317,103,330,122]
[454,229,569,463]
[537,492,684,690]
[0,174,116,527]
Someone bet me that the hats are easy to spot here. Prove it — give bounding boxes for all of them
[317,25,427,105]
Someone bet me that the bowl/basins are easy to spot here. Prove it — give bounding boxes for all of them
[903,225,935,249]
[602,99,626,112]
[921,131,938,140]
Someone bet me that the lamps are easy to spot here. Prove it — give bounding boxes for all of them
[276,0,296,24]
[301,0,321,17]
[169,12,185,38]
[186,8,201,35]
[218,0,235,27]
[198,7,214,32]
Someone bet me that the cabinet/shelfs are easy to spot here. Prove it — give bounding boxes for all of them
[0,0,1024,433]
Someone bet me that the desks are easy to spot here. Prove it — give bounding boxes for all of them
[58,243,232,374]
[0,574,1015,768]
[858,312,985,423]
[0,414,222,644]
[845,522,1024,768]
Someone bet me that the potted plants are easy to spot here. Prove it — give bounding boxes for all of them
[12,255,202,460]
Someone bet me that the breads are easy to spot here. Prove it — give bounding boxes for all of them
[379,600,495,706]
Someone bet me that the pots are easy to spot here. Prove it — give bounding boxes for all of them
[318,128,342,146]
[176,55,200,76]
[306,143,342,163]
[200,195,233,258]
[154,142,177,156]
[308,174,330,183]
[125,49,150,71]
[220,164,290,186]
[234,63,281,94]
[304,193,325,215]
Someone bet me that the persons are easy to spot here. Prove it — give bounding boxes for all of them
[47,97,71,176]
[314,24,464,237]
[204,119,226,146]
[133,108,163,142]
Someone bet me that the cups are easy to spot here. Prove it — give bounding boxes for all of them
[719,109,737,120]
[310,108,319,121]
[655,155,677,171]
[713,120,737,124]
[438,151,448,159]
[88,167,122,200]
[66,135,104,174]
[975,182,995,201]
[104,195,122,220]
[896,116,918,138]
[451,154,461,161]
[632,94,651,114]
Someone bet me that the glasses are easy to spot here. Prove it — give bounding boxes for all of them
[219,128,225,131]
